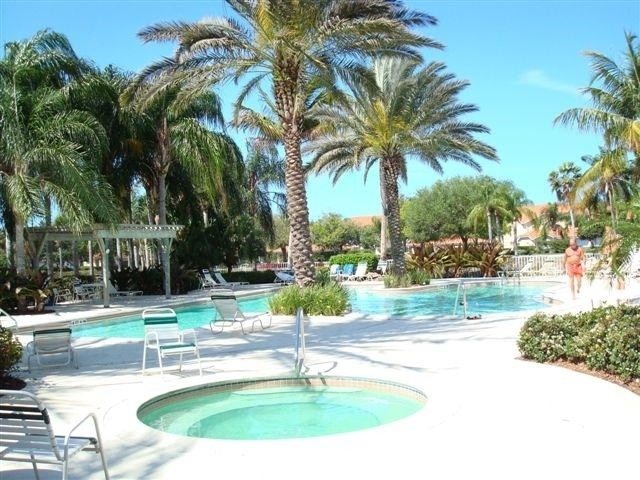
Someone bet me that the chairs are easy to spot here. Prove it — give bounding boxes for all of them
[0,390,109,480]
[143,308,203,375]
[27,327,79,374]
[497,262,552,278]
[197,268,248,290]
[274,260,372,285]
[52,280,143,305]
[210,289,272,335]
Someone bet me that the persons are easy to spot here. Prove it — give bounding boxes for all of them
[562,239,586,299]
